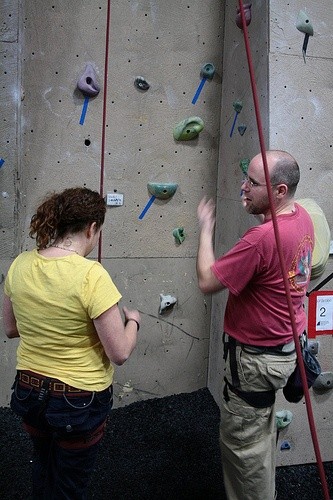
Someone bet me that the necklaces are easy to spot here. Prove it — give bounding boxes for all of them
[49,244,78,255]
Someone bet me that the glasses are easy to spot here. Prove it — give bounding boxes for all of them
[243,175,276,187]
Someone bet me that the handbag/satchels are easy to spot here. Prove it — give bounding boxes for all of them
[282,350,320,403]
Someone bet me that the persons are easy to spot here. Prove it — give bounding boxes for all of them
[2,188,140,500]
[196,151,315,500]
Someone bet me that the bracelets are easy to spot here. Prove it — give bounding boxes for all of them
[125,319,140,332]
